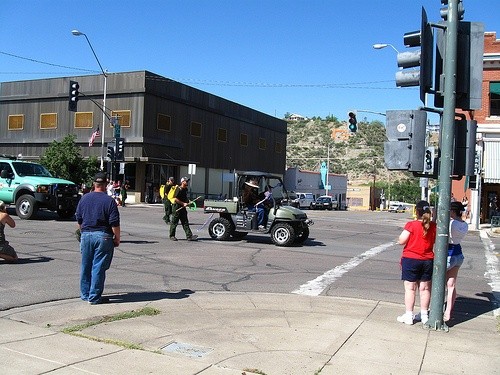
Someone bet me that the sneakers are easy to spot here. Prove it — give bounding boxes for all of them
[416,313,429,323]
[397,313,415,324]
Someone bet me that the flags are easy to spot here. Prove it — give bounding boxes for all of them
[88,127,100,147]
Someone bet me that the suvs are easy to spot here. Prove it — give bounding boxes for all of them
[282,192,317,210]
[0,153,82,222]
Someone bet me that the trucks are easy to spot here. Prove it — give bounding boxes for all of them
[203,168,315,247]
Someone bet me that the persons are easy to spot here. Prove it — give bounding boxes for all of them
[75,172,120,304]
[169,177,198,241]
[144,183,161,203]
[397,200,437,325]
[0,200,18,262]
[416,202,467,322]
[106,177,128,207]
[451,193,469,220]
[162,177,175,225]
[242,179,275,229]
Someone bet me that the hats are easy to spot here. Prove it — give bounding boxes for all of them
[94,173,107,183]
[181,177,191,182]
[169,177,174,181]
[265,185,274,189]
[245,180,260,189]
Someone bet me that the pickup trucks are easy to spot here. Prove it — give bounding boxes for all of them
[315,195,337,211]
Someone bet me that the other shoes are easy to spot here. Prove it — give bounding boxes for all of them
[96,297,109,304]
[188,235,198,240]
[170,237,177,241]
[258,225,264,229]
[162,217,172,225]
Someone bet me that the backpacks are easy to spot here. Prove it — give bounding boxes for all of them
[160,185,167,200]
[167,184,182,204]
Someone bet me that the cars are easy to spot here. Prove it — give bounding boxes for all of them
[389,204,403,213]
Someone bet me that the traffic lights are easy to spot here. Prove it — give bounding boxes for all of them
[107,142,116,162]
[383,110,425,173]
[115,137,125,159]
[69,81,80,112]
[347,110,358,133]
[422,145,435,175]
[440,0,465,21]
[396,6,433,106]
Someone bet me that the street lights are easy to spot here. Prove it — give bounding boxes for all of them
[71,29,108,172]
